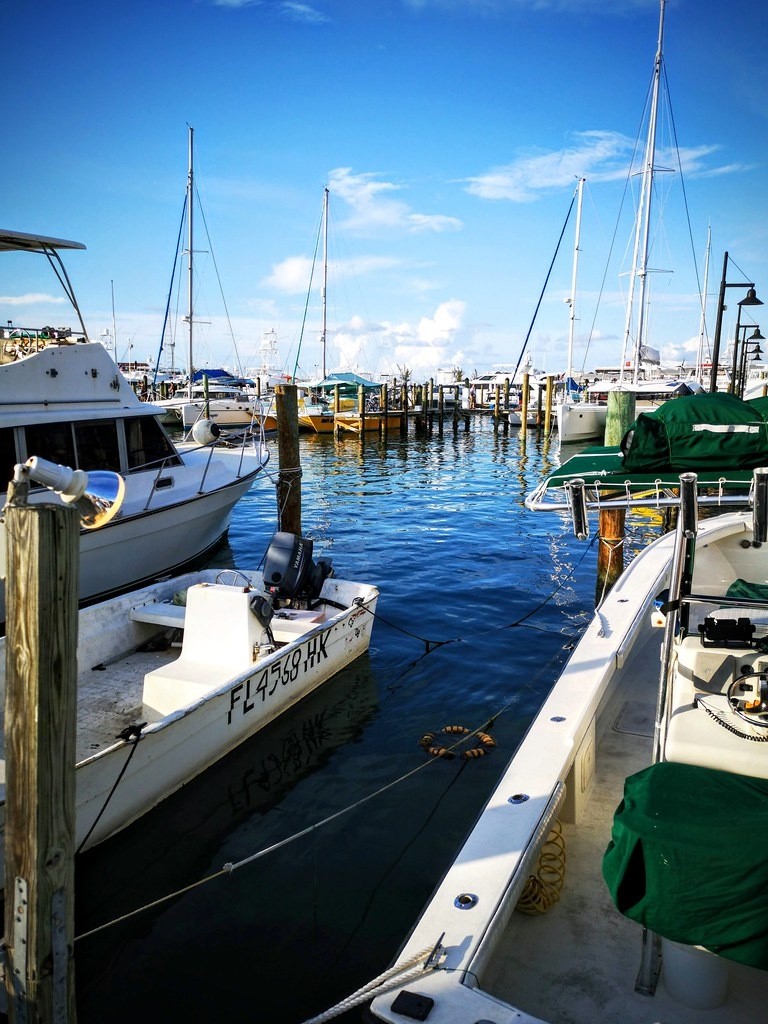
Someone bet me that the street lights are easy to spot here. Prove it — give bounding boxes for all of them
[710,249,766,401]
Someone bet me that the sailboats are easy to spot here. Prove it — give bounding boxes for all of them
[427,0,712,442]
[129,122,402,430]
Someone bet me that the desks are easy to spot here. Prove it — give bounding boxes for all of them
[601,760,768,997]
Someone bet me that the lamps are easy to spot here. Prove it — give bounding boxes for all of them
[24,455,125,530]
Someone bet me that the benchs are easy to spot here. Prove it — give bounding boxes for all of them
[129,602,321,642]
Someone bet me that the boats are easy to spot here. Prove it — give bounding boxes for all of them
[0,230,380,888]
[370,467,768,1024]
